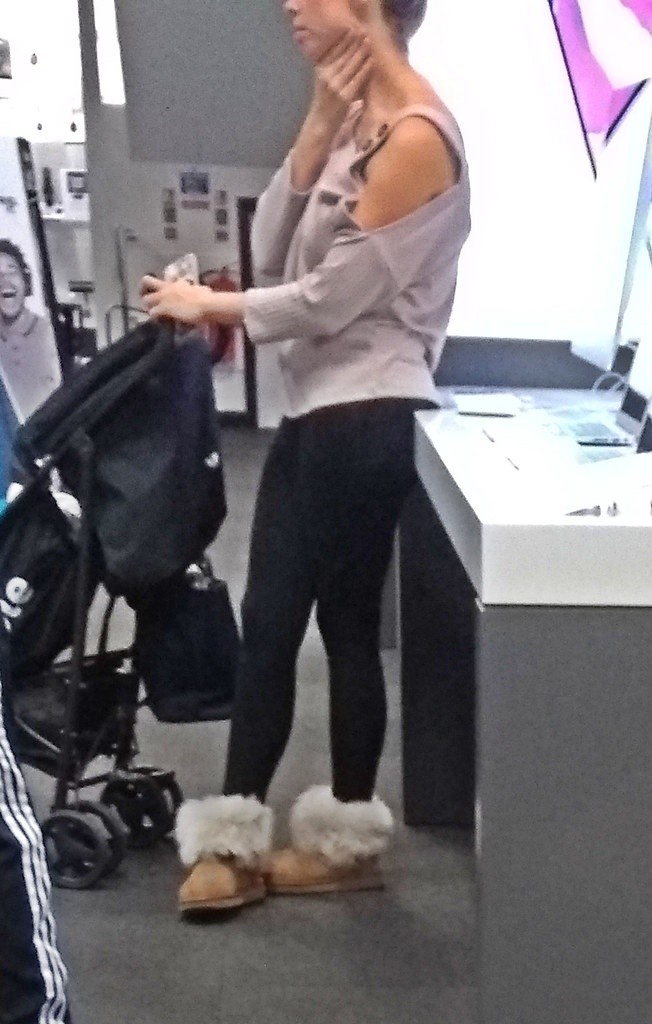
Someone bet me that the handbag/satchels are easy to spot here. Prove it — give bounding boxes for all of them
[134,558,240,723]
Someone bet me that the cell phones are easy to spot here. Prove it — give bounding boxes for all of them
[164,253,199,334]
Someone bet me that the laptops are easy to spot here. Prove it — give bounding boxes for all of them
[558,323,652,445]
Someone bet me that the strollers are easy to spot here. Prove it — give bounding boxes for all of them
[0,284,239,890]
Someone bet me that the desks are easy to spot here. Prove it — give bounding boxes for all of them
[394,379,652,1022]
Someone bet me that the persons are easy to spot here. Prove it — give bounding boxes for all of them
[0,239,62,424]
[138,2,473,915]
[0,682,76,1024]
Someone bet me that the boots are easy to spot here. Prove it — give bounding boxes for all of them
[175,795,272,914]
[258,786,395,892]
